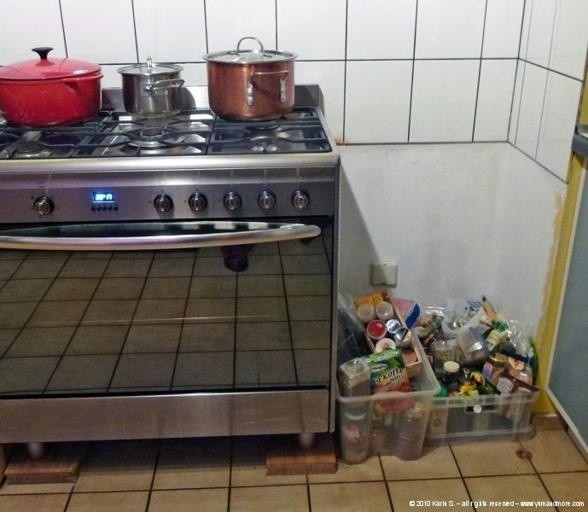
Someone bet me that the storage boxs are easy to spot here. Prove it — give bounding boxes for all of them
[424,301,542,448]
[325,297,442,476]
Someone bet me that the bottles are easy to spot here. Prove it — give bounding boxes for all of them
[338,294,441,465]
[441,361,460,391]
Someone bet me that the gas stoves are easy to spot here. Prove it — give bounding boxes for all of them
[1,109,343,170]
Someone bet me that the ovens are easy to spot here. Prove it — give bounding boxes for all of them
[0,171,342,456]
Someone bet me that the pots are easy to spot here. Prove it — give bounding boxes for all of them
[201,36,296,121]
[1,44,104,125]
[115,58,186,118]
